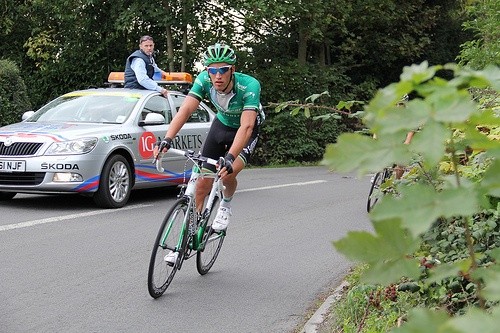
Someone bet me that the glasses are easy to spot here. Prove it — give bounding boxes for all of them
[207,65,232,75]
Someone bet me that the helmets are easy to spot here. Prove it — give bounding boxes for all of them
[203,43,237,66]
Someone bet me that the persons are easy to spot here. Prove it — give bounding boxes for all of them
[123,35,169,97]
[370,93,416,193]
[153,43,266,263]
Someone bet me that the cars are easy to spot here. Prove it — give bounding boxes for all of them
[0,70,217,210]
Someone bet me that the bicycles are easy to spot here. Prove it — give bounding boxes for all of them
[366,163,398,213]
[148,140,228,299]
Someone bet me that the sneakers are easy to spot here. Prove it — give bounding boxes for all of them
[164,246,181,271]
[212,201,232,230]
